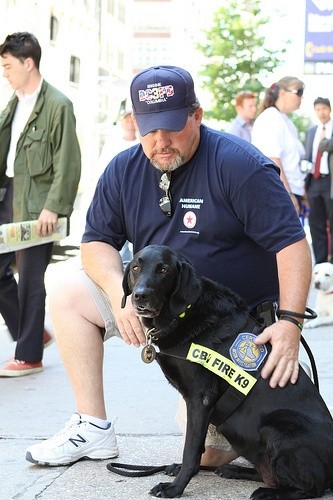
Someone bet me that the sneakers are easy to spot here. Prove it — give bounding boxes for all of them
[44,329,55,348]
[0,358,44,377]
[25,412,119,466]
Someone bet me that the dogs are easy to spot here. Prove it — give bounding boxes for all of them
[302,261,333,329]
[118,245,333,500]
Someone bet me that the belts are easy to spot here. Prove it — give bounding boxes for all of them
[310,173,328,178]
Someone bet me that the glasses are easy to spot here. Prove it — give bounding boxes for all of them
[158,170,172,218]
[284,87,304,97]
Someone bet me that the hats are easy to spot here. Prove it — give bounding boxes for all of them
[130,65,196,137]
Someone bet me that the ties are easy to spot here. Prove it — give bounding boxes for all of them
[313,127,326,180]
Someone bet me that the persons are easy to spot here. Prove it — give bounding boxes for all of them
[0,32,79,376]
[95,97,139,269]
[23,67,313,468]
[223,76,333,262]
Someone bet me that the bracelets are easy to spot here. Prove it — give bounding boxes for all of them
[278,316,303,331]
